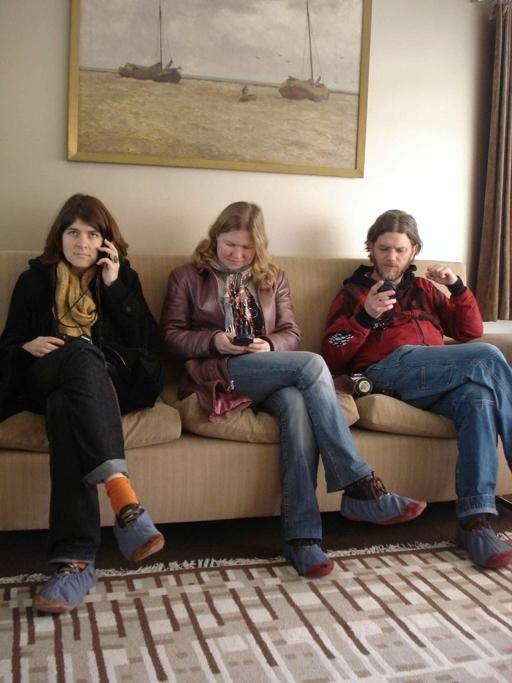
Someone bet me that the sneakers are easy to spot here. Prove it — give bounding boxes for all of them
[340,479,426,525]
[282,538,333,578]
[113,504,165,562]
[33,562,96,614]
[458,517,512,568]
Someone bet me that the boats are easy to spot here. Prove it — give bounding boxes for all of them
[240,84,257,103]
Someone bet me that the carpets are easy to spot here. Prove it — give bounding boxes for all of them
[0,529,512,683]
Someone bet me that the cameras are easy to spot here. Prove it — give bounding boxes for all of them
[351,373,374,394]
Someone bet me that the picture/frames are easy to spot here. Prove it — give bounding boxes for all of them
[67,0,375,178]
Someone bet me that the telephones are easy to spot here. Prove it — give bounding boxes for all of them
[88,236,113,290]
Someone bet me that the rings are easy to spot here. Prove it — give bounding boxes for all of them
[110,255,118,263]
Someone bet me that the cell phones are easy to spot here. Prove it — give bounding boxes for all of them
[377,282,396,307]
[99,245,110,269]
[232,333,255,348]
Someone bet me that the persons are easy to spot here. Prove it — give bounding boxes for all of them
[322,210,512,568]
[159,202,428,578]
[0,193,165,614]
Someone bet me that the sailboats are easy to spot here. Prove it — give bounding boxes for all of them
[278,1,330,100]
[117,1,183,84]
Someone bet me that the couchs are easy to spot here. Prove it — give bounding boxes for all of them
[1,250,512,532]
